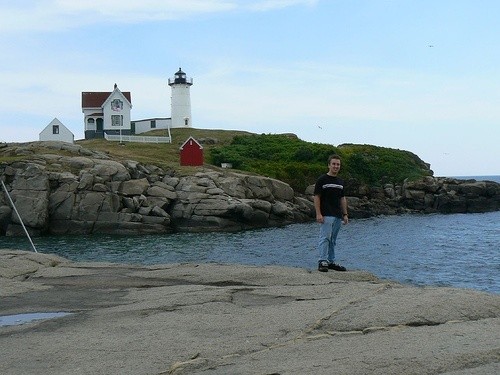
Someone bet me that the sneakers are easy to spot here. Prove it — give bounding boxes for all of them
[318,261,328,271]
[328,261,346,271]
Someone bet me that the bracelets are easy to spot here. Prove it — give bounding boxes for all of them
[343,214,347,216]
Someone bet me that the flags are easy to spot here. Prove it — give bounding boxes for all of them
[113,104,121,112]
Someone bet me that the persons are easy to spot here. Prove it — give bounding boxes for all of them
[312,155,349,273]
